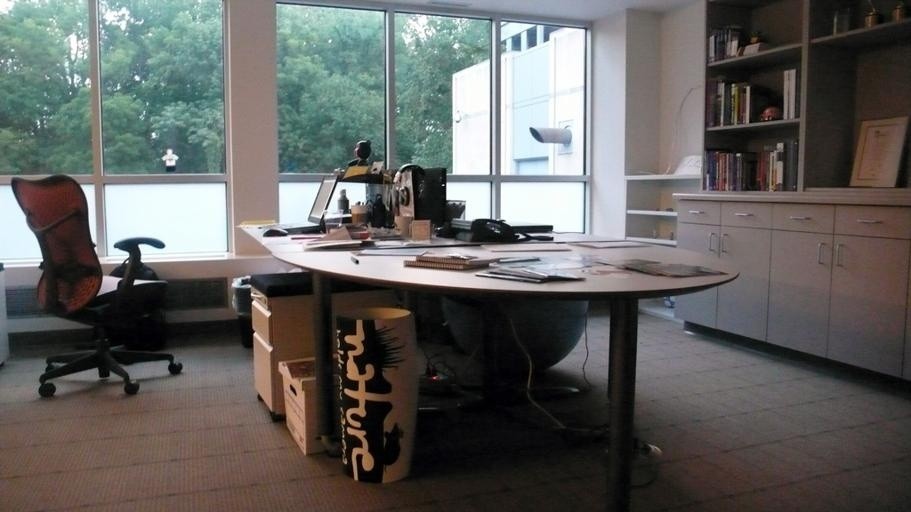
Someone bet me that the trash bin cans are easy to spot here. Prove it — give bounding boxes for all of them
[331,307,419,484]
[230,275,252,347]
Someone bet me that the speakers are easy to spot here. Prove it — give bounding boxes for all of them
[398,167,447,221]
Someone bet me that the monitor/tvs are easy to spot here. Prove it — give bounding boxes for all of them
[307,175,338,231]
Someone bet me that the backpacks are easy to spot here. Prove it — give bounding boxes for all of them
[105,257,166,351]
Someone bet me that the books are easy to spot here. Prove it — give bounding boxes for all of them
[403,230,729,285]
[305,229,378,251]
[702,27,801,192]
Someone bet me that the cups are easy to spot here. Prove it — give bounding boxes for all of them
[351,205,368,224]
[324,209,344,235]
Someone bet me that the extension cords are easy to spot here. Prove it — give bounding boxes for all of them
[419,375,450,385]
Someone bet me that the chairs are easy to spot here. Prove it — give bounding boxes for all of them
[10,175,183,397]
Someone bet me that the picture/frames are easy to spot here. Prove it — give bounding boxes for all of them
[849,117,908,190]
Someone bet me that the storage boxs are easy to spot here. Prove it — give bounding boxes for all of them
[278,357,321,456]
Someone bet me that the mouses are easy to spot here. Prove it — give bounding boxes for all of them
[262,227,288,237]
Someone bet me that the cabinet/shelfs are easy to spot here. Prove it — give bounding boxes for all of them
[673,200,766,344]
[767,201,910,376]
[806,2,910,201]
[248,273,397,421]
[704,1,807,194]
[626,173,700,324]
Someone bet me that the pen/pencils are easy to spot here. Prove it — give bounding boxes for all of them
[291,237,321,240]
[351,256,358,264]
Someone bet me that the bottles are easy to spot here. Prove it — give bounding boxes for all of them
[338,189,349,213]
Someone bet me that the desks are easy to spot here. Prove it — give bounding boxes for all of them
[240,214,739,504]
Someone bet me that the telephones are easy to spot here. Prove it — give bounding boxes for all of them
[451,218,519,243]
